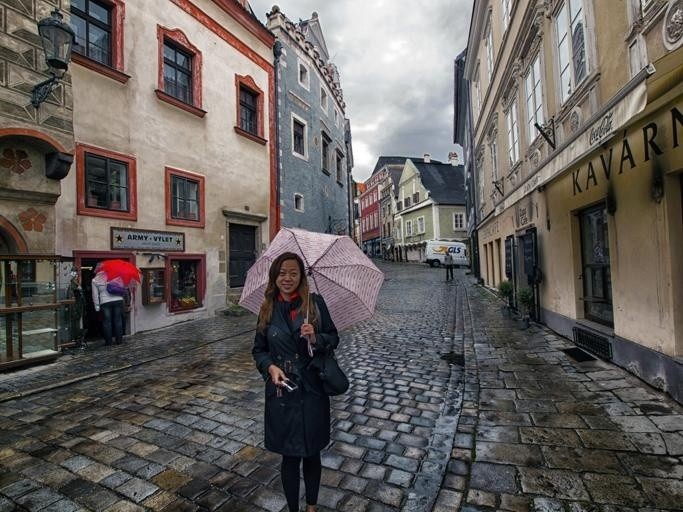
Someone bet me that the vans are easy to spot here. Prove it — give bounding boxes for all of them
[425,241,467,267]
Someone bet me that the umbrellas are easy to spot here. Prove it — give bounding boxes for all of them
[238,227,385,323]
[94,259,143,287]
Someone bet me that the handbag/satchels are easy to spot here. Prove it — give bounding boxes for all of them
[307,343,349,398]
[106,281,130,297]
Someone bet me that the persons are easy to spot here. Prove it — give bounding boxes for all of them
[444,250,453,281]
[252,252,339,512]
[92,271,131,345]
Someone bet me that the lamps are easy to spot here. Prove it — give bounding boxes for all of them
[28,7,76,110]
